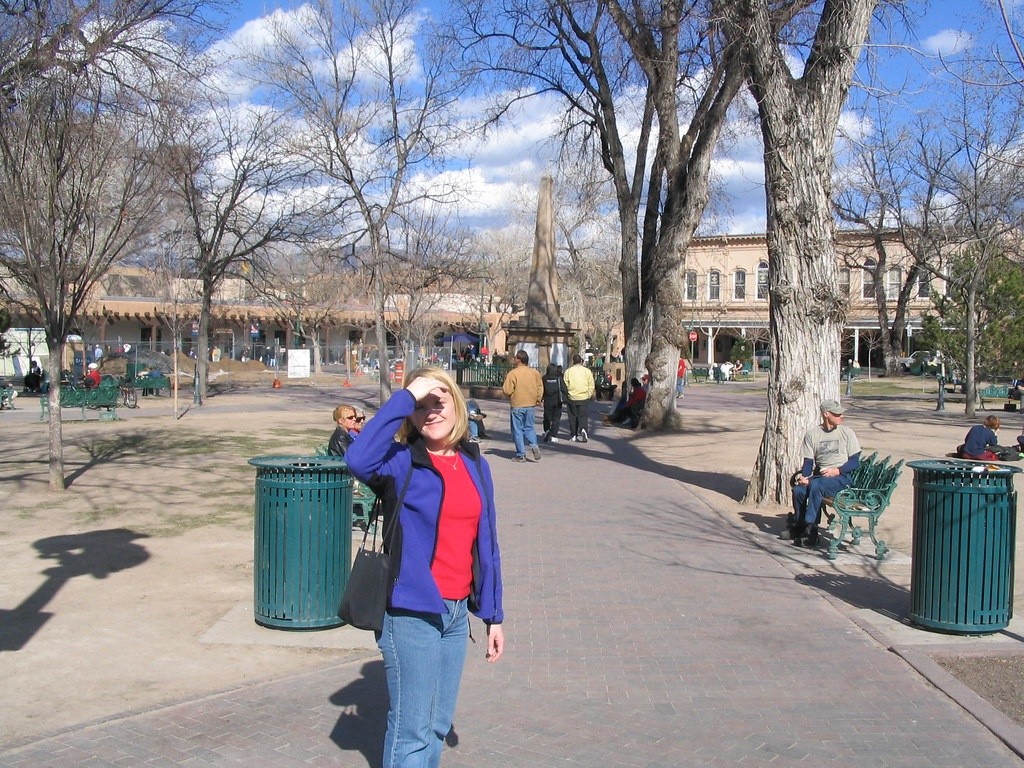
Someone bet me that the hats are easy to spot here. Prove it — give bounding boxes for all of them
[88,363,98,369]
[820,399,847,414]
[640,374,649,380]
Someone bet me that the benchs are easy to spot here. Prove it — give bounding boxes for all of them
[937,372,967,394]
[692,367,725,384]
[315,441,379,535]
[732,370,749,380]
[795,449,905,561]
[0,383,17,409]
[978,385,1023,409]
[127,374,173,397]
[41,384,119,421]
[841,367,862,379]
[762,359,771,371]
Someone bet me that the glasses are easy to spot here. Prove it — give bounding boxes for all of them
[355,416,365,423]
[343,415,356,420]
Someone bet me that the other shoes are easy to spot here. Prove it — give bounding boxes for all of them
[550,436,558,442]
[542,430,553,442]
[478,412,486,417]
[568,435,577,442]
[602,415,612,426]
[677,394,684,399]
[478,433,489,440]
[469,413,483,420]
[468,436,482,443]
[794,524,819,546]
[779,519,808,540]
[511,454,526,462]
[532,446,542,459]
[580,428,588,442]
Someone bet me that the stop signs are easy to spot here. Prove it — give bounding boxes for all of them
[689,332,697,341]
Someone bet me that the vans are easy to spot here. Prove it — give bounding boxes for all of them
[748,350,771,368]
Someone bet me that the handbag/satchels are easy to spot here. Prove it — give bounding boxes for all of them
[557,377,565,402]
[338,461,415,632]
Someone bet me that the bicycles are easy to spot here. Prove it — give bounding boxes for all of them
[110,376,141,409]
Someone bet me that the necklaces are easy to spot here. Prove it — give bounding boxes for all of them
[433,453,460,471]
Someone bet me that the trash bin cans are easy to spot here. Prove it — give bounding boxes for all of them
[743,359,753,372]
[248,452,355,632]
[906,457,1023,635]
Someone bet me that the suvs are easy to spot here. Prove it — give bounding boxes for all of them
[898,350,945,373]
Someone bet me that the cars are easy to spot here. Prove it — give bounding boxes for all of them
[339,343,451,372]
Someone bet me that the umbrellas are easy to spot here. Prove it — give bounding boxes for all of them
[436,333,484,351]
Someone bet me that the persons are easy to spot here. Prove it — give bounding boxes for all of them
[23,362,40,393]
[713,359,743,380]
[0,381,18,410]
[677,355,686,399]
[329,404,383,526]
[781,400,862,548]
[94,343,103,363]
[188,345,215,360]
[1013,377,1024,414]
[542,364,568,442]
[957,415,1000,460]
[602,374,649,426]
[502,350,544,462]
[961,371,966,392]
[139,365,165,395]
[563,355,595,443]
[339,340,446,367]
[595,369,614,401]
[84,362,100,389]
[625,378,646,428]
[464,343,498,365]
[231,343,333,366]
[344,367,504,768]
